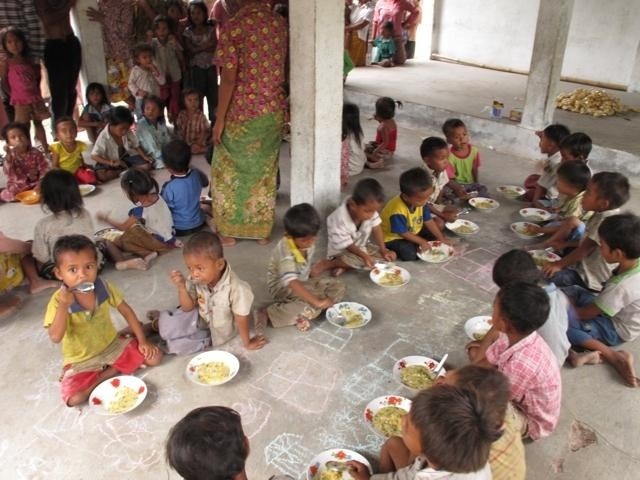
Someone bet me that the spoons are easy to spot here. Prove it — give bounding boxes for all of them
[68,283,95,295]
[181,275,200,284]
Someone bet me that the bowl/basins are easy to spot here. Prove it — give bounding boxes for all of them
[16,189,40,205]
[324,301,373,330]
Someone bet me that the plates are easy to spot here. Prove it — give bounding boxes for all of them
[94,228,125,238]
[186,350,241,388]
[416,183,563,273]
[369,265,411,289]
[305,355,447,479]
[464,316,495,342]
[78,184,96,196]
[88,375,148,417]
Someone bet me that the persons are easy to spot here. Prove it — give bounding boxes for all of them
[166,405,252,480]
[1,2,291,320]
[458,122,639,442]
[41,235,164,406]
[254,202,346,332]
[344,382,494,480]
[345,0,422,80]
[436,369,530,480]
[306,96,489,277]
[118,231,267,356]
